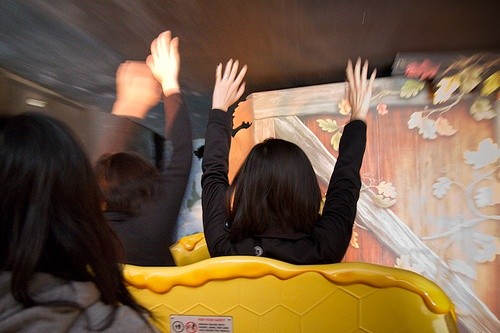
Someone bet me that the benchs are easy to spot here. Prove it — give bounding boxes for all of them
[115,256,459,333]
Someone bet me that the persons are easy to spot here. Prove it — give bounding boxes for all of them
[201,57,377,267]
[90,30,192,268]
[0,111,157,333]
